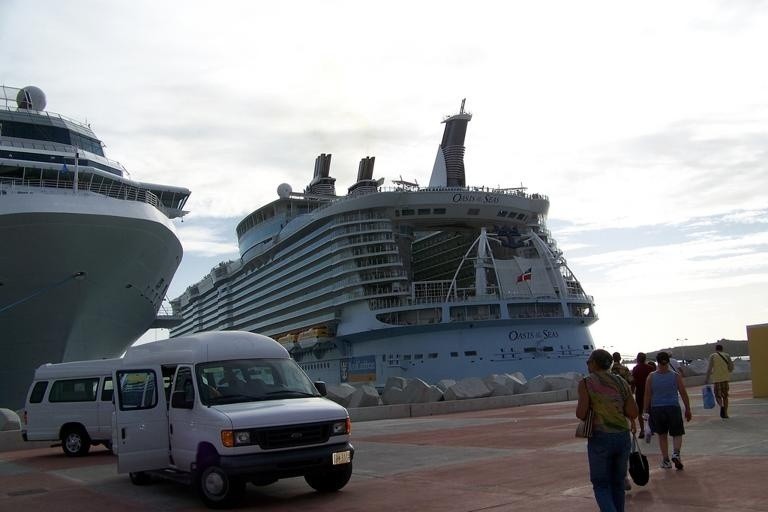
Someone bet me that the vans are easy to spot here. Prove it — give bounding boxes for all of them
[111,330,355,508]
[23,358,123,456]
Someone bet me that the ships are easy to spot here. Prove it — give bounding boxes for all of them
[167,98,598,393]
[1,84,191,414]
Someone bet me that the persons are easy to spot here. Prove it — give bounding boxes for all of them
[575,349,640,512]
[704,344,734,418]
[611,350,692,470]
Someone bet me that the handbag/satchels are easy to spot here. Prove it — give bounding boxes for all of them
[629,452,649,486]
[703,386,715,409]
[576,408,593,438]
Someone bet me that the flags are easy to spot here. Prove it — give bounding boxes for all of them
[516,267,531,282]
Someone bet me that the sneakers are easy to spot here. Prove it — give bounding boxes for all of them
[720,406,728,418]
[660,460,671,468]
[671,453,683,469]
